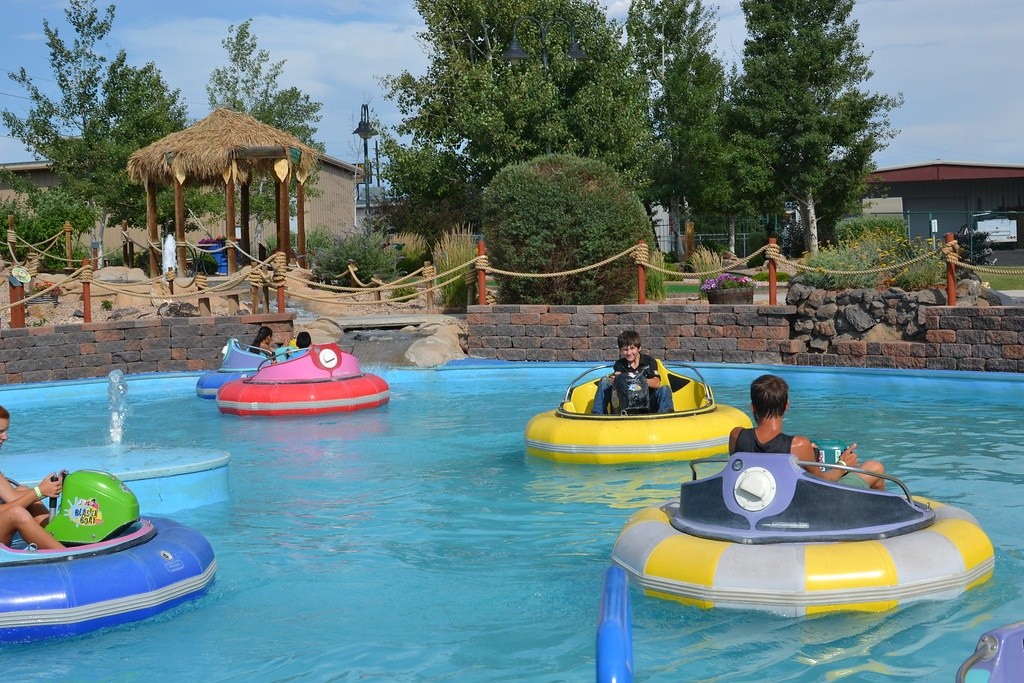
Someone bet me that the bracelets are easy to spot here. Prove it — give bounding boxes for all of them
[839,461,847,473]
[34,487,42,500]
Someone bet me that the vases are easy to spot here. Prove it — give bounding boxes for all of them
[707,288,753,304]
[204,241,242,275]
[25,294,59,307]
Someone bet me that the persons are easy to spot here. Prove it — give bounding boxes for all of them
[728,375,885,490]
[0,406,66,549]
[271,332,311,364]
[250,326,275,356]
[592,331,674,415]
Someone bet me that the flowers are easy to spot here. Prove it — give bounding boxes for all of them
[199,236,221,244]
[701,273,753,293]
[24,281,64,297]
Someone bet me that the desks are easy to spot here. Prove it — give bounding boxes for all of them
[187,244,218,276]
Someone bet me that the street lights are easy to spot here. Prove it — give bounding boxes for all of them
[353,103,379,240]
